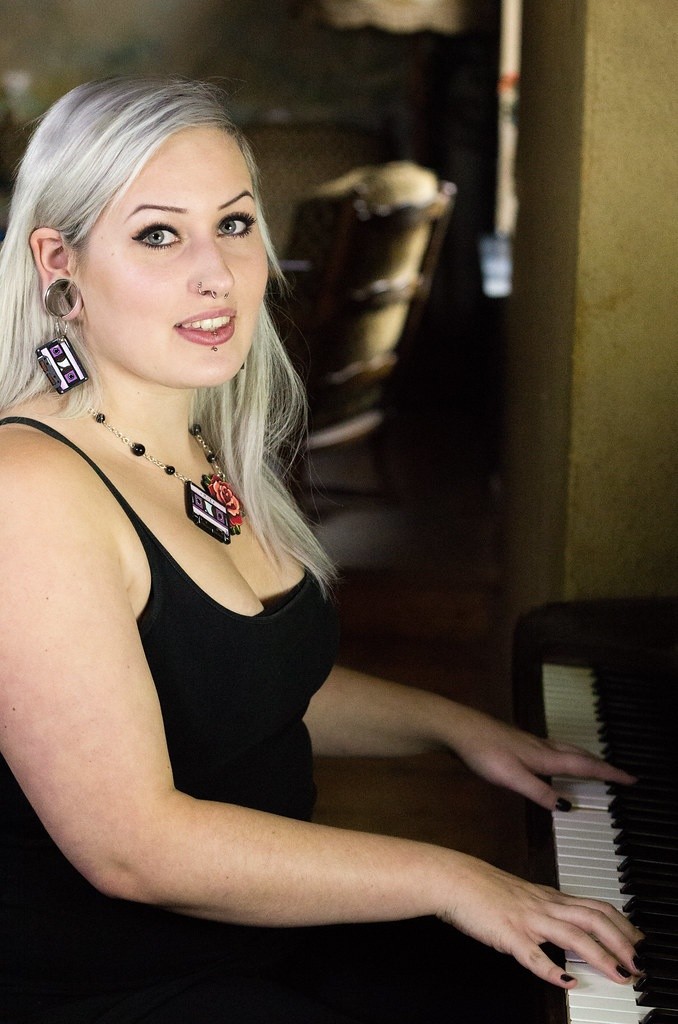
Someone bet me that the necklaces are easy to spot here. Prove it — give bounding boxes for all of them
[65,380,244,545]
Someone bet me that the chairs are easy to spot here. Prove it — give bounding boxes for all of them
[267,158,458,499]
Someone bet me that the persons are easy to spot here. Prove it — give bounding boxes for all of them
[1,66,651,1024]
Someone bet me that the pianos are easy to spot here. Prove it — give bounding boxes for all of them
[503,593,677,1024]
